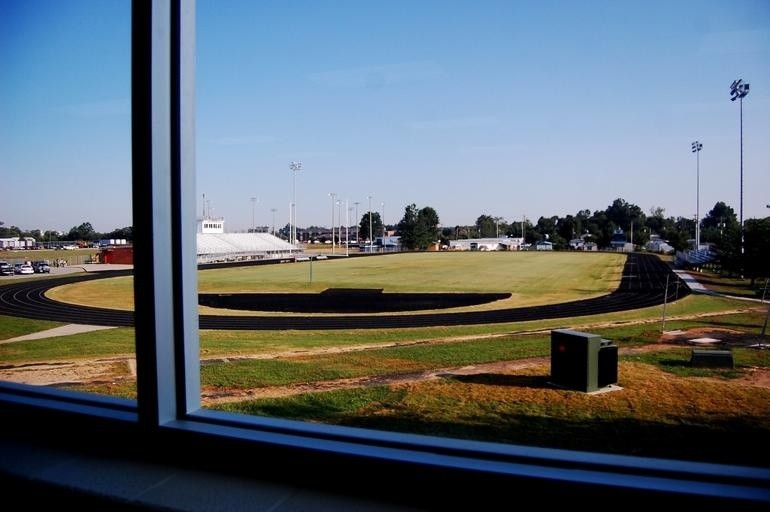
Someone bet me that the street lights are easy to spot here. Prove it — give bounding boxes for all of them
[198,158,387,259]
[690,139,703,249]
[729,78,751,283]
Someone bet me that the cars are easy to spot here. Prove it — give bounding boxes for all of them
[0,260,53,276]
